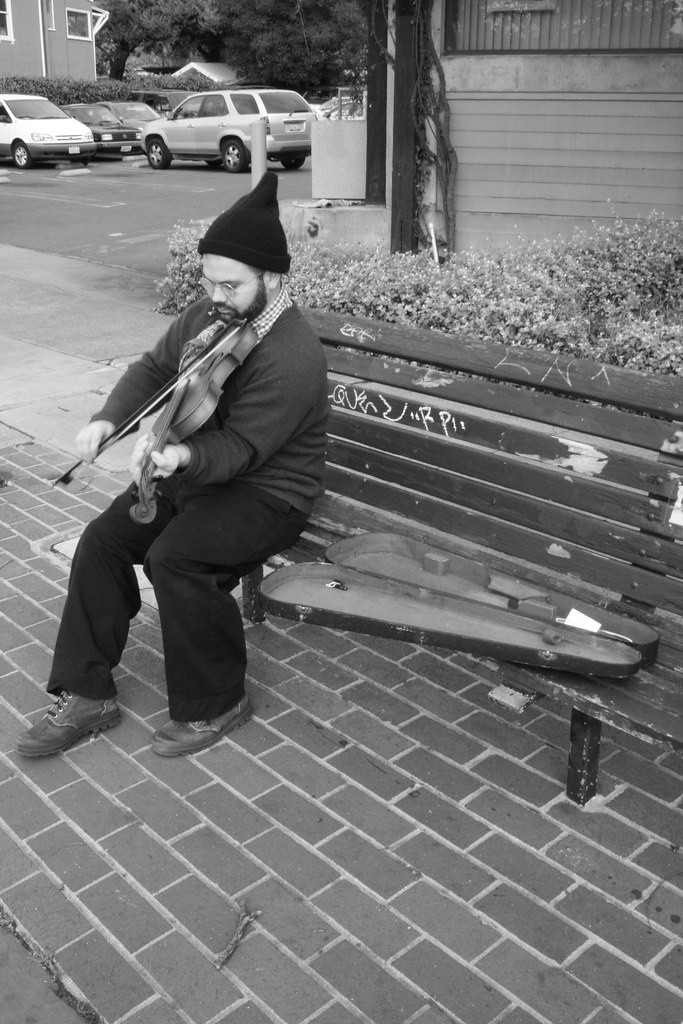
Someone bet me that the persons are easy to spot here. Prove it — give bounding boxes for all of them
[14,172,331,759]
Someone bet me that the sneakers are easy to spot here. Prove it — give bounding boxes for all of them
[15,690,120,757]
[152,691,253,757]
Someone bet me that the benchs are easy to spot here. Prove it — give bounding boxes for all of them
[242,306,683,805]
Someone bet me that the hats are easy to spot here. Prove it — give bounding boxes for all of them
[197,172,290,272]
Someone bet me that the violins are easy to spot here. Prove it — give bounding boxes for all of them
[129,319,260,527]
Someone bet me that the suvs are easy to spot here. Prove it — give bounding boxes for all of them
[125,90,208,119]
[141,87,318,173]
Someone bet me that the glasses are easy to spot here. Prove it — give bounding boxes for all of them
[200,275,260,298]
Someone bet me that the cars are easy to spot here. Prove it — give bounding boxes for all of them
[94,101,161,139]
[0,94,98,167]
[319,98,364,117]
[59,104,145,160]
